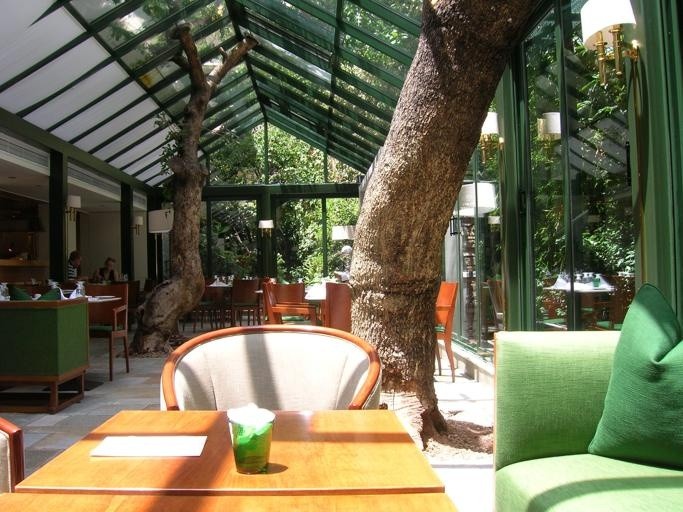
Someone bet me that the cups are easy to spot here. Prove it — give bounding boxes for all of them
[225,405,275,477]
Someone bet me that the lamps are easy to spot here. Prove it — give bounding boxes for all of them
[131,216,144,236]
[64,193,82,222]
[536,108,565,152]
[332,224,356,273]
[478,111,505,166]
[453,181,500,344]
[148,209,176,282]
[578,1,642,90]
[258,218,274,237]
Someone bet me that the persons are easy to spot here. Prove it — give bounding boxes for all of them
[484,259,502,284]
[65,250,92,284]
[335,243,354,275]
[93,257,120,282]
